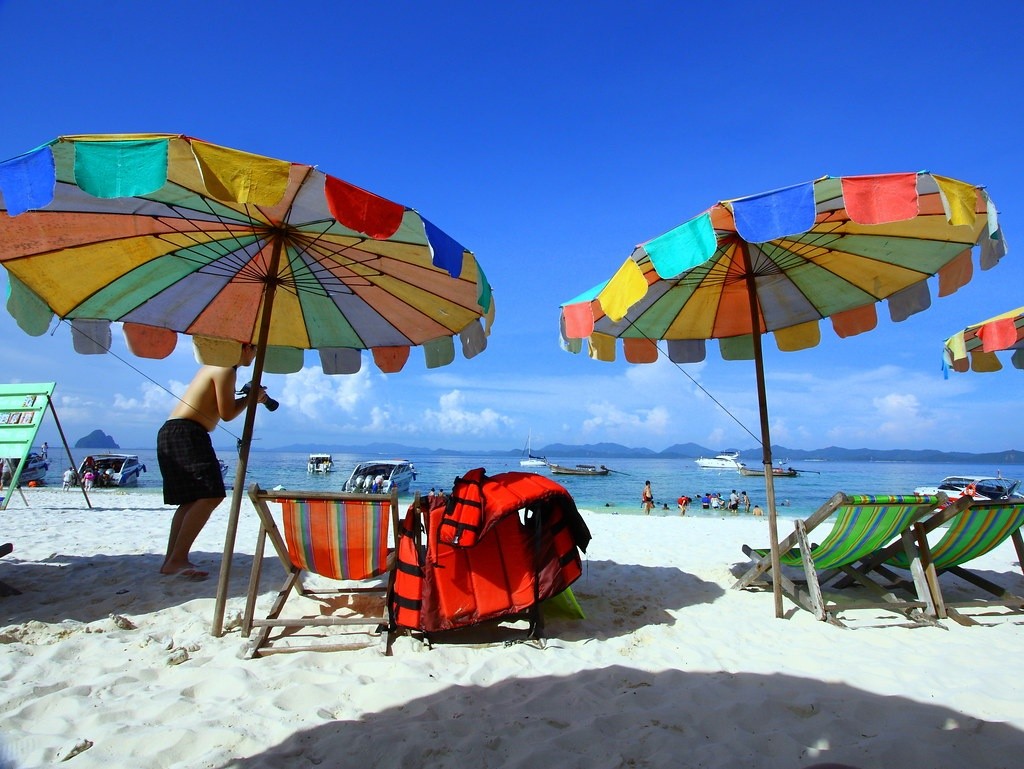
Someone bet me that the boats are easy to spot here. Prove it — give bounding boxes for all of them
[740,468,797,477]
[346,459,420,494]
[693,451,746,468]
[913,467,1024,513]
[76,453,146,487]
[0,453,50,486]
[549,463,610,475]
[218,459,228,478]
[308,453,334,474]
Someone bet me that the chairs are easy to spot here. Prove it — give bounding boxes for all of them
[242,484,549,660]
[732,492,1024,630]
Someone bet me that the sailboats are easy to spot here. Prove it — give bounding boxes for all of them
[519,428,546,467]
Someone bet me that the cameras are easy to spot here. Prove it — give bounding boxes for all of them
[244,381,279,412]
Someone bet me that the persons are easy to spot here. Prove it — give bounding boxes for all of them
[643,480,751,517]
[83,465,94,491]
[62,466,75,491]
[41,442,48,460]
[0,458,16,491]
[753,505,764,516]
[157,343,268,577]
[438,489,445,496]
[428,488,436,496]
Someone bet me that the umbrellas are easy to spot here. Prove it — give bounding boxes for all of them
[558,170,1008,618]
[942,306,1024,380]
[0,133,496,638]
